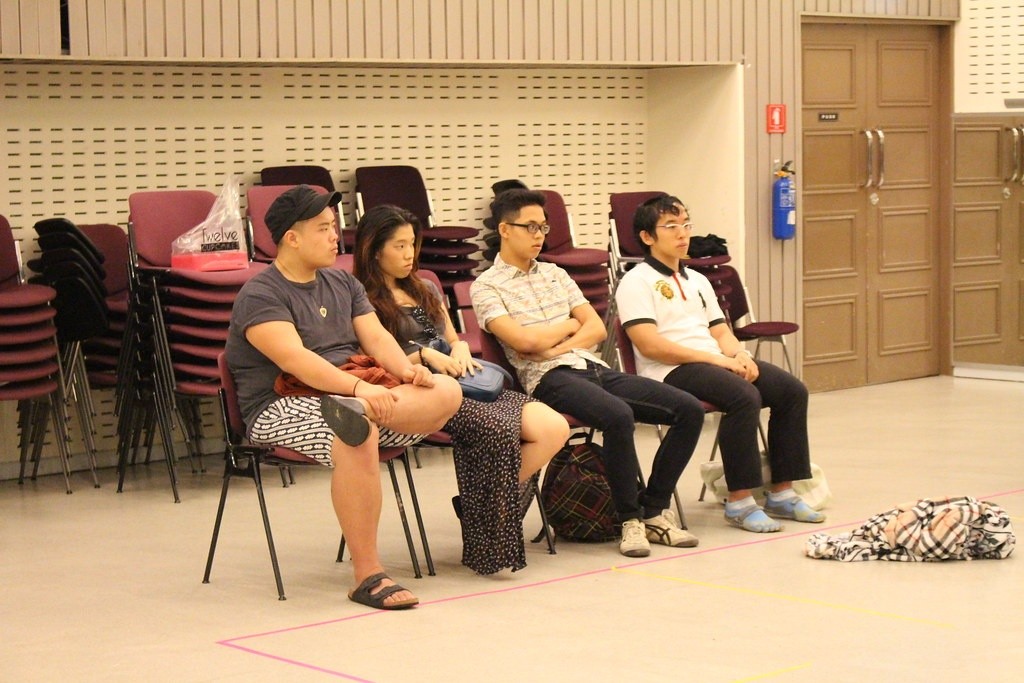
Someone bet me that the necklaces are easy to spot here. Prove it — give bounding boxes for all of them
[277,257,327,318]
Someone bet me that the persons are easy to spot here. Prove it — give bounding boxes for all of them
[353,203,569,576]
[615,195,825,532]
[468,190,705,557]
[226,183,463,608]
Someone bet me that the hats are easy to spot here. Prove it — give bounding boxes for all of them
[264,183,343,244]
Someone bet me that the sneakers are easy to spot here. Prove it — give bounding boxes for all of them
[640,509,699,548]
[619,521,651,556]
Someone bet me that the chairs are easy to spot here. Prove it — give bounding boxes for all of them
[0,169,802,608]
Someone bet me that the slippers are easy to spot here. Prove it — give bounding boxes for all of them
[348,572,419,609]
[321,393,373,447]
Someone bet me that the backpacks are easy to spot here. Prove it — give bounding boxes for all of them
[541,432,644,544]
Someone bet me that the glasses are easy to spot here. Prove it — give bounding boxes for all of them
[413,306,437,339]
[646,223,694,236]
[505,222,551,234]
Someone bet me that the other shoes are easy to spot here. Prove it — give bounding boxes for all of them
[452,495,463,519]
[724,504,785,532]
[763,494,827,523]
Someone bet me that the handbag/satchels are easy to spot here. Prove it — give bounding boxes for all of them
[429,338,514,402]
[274,354,401,398]
[699,448,833,511]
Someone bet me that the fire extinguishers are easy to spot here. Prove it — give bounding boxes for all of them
[773,159,796,240]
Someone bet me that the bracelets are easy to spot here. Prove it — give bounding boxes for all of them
[353,379,361,396]
[419,346,425,366]
